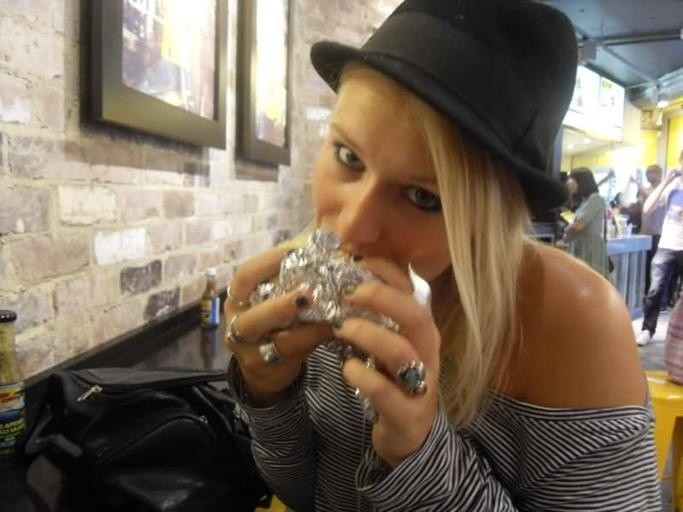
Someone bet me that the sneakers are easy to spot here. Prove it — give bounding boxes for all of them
[636,330,652,346]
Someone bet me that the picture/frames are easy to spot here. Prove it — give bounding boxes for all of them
[242,1,290,165]
[90,0,226,149]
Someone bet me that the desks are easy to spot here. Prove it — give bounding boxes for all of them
[22,286,229,423]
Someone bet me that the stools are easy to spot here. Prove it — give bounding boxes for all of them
[644,369,683,512]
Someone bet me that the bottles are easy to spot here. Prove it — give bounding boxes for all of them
[199,266,221,330]
[200,328,217,371]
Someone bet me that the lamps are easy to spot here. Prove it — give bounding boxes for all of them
[580,39,600,65]
[645,85,668,108]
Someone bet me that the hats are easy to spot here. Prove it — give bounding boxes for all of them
[311,1,576,210]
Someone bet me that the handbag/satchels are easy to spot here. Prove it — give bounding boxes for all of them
[23,368,273,512]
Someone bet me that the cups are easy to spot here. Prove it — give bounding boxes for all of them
[608,225,615,237]
[619,213,629,236]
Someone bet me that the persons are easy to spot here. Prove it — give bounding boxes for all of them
[662,291,683,387]
[634,149,682,348]
[562,166,609,284]
[217,1,664,512]
[625,164,664,295]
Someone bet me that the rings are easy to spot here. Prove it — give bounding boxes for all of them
[258,331,282,365]
[225,285,250,307]
[395,360,428,397]
[225,314,250,346]
